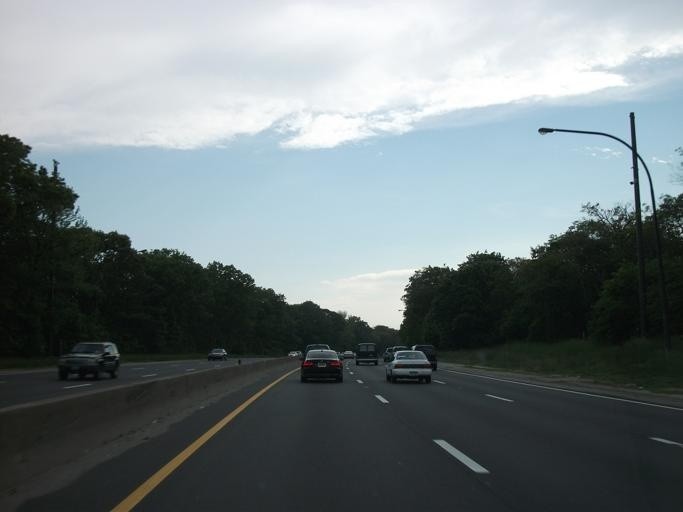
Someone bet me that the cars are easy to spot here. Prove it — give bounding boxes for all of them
[284,340,437,385]
[207,348,229,363]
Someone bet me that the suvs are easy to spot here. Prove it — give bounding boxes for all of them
[56,340,121,379]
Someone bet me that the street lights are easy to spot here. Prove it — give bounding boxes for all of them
[536,126,671,353]
